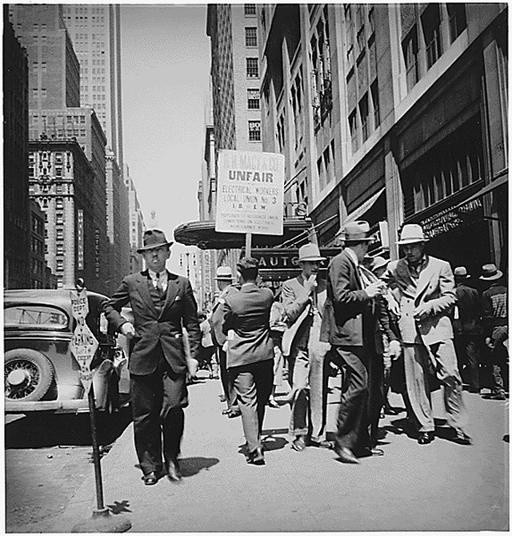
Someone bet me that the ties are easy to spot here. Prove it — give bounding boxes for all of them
[156,273,163,298]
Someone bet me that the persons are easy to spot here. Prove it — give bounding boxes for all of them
[221,259,274,461]
[320,222,401,463]
[198,266,286,417]
[447,266,482,393]
[363,224,472,442]
[478,265,508,399]
[281,243,335,450]
[105,228,200,487]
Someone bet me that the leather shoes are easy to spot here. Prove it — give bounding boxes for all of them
[222,410,472,462]
[166,459,181,482]
[144,472,157,484]
[269,400,280,408]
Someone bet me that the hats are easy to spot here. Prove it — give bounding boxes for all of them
[214,221,503,280]
[137,230,173,254]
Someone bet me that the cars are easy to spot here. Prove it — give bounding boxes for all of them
[3,288,132,413]
